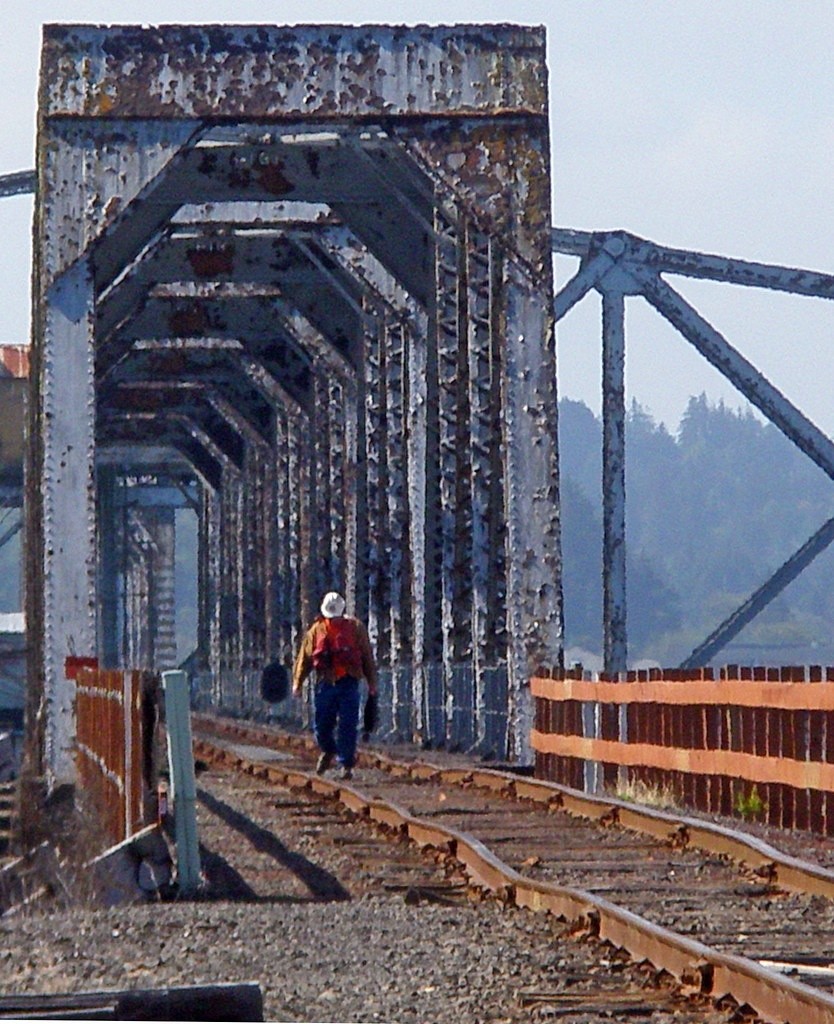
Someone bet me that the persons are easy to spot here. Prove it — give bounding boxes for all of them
[290,592,379,785]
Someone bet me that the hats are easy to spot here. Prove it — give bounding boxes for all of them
[319,592,345,619]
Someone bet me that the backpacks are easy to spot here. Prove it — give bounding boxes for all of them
[313,619,360,679]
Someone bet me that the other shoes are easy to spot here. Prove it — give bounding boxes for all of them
[334,766,353,779]
[316,750,334,775]
